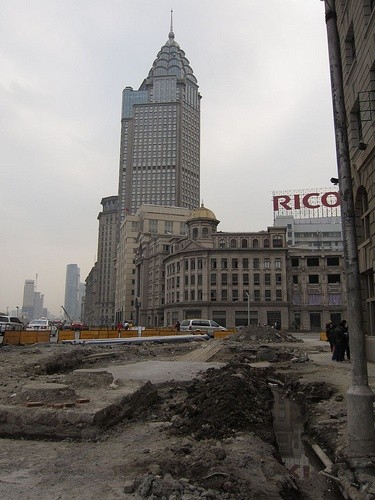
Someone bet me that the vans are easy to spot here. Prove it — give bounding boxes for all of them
[180,319,228,337]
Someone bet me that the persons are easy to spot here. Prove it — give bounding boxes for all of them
[124,321,133,330]
[111,321,123,330]
[326,319,350,362]
[175,321,180,331]
[274,321,280,330]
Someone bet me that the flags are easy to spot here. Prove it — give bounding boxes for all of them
[126,208,129,212]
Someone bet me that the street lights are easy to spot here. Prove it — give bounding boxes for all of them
[244,290,253,326]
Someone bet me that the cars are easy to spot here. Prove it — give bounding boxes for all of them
[0,315,25,334]
[26,318,58,337]
[52,319,90,331]
